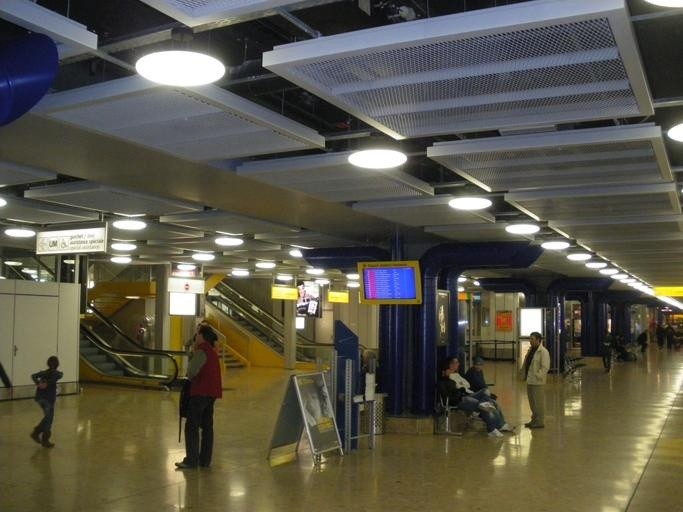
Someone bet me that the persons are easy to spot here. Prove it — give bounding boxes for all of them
[636,329,649,353]
[174,324,224,470]
[437,360,506,439]
[297,377,328,426]
[463,355,508,422]
[29,354,65,449]
[443,354,516,439]
[294,281,316,313]
[598,327,617,372]
[664,322,677,350]
[616,334,627,363]
[655,322,665,351]
[184,319,211,360]
[0,364,15,402]
[519,330,551,429]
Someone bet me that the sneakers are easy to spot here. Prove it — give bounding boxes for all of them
[29,433,41,443]
[499,423,514,432]
[43,441,55,447]
[487,428,504,438]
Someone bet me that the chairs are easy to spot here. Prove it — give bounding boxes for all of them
[436,384,507,436]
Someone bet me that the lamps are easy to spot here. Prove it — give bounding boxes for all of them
[505,215,540,234]
[135,25,227,87]
[448,184,493,210]
[348,133,407,169]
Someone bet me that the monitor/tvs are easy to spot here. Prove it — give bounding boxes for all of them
[356,260,425,305]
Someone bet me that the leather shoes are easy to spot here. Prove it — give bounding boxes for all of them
[175,460,191,468]
[524,420,534,426]
[530,423,544,428]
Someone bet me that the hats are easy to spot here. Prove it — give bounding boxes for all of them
[473,355,485,365]
[198,324,218,346]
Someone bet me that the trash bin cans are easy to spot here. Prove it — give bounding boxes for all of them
[353,393,388,435]
